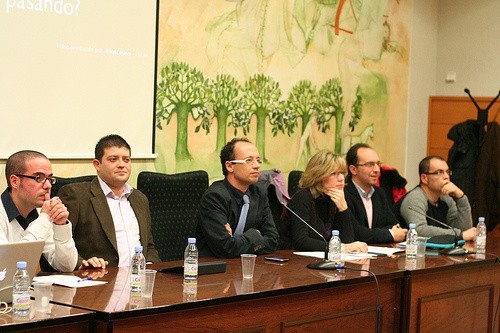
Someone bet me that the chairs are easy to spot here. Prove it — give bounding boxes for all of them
[51,175,97,198]
[288,171,304,197]
[137,170,210,261]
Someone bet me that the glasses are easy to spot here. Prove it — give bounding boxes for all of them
[230,158,263,166]
[355,162,381,167]
[422,169,452,176]
[15,174,57,185]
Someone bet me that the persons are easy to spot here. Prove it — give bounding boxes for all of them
[80,270,108,280]
[0,150,78,273]
[400,155,477,244]
[277,149,369,254]
[56,134,161,269]
[194,136,279,259]
[344,144,409,244]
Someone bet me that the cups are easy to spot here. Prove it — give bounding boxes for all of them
[138,269,157,298]
[416,259,426,271]
[240,279,254,294]
[32,280,53,313]
[417,238,427,258]
[140,298,153,309]
[240,253,258,279]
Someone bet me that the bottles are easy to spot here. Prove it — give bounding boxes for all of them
[405,223,418,259]
[474,217,487,253]
[183,237,199,280]
[130,244,147,292]
[328,230,342,265]
[183,278,197,301]
[128,292,143,311]
[12,261,31,310]
[404,257,416,270]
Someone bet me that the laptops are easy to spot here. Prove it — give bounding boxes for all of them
[0,241,45,304]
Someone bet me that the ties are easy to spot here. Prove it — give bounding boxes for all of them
[233,194,250,235]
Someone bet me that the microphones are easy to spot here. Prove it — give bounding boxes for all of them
[281,202,339,270]
[408,208,468,256]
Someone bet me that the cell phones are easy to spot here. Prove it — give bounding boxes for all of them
[265,256,288,261]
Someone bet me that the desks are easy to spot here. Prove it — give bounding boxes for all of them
[0,237,500,333]
